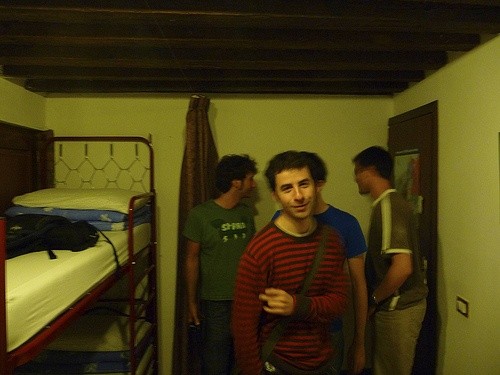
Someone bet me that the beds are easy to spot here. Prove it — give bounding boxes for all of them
[1,137,158,374]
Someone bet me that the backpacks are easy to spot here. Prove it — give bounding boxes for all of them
[6,214,100,259]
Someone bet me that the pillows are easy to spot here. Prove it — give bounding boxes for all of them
[49,314,153,352]
[12,187,152,214]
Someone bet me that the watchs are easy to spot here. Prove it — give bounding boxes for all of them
[369,293,379,308]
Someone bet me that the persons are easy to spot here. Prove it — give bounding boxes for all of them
[271,151,369,375]
[231,150,354,375]
[182,153,259,375]
[350,145,429,375]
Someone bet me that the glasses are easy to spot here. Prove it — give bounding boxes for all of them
[354,159,379,175]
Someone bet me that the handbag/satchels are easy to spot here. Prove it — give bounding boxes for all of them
[230,362,240,375]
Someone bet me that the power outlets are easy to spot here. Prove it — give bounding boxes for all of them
[456,295,470,318]
